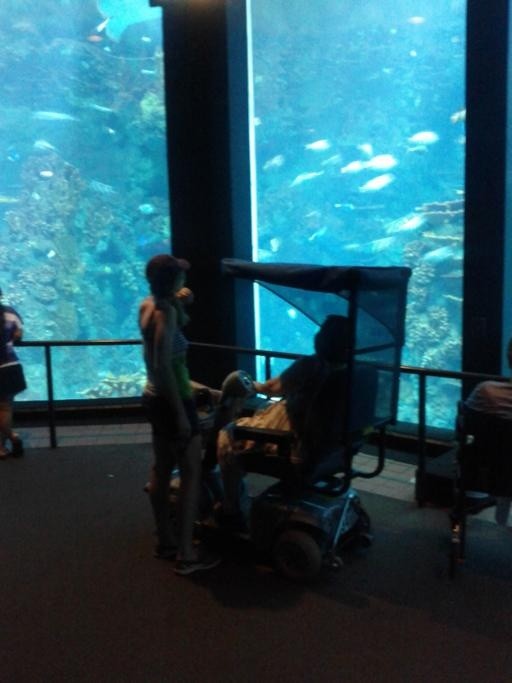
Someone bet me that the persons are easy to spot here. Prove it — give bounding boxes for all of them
[0,289,27,458]
[444,340,512,518]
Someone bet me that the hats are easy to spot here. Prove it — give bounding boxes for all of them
[146,252,191,281]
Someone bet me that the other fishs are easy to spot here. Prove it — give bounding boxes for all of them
[255,109,468,321]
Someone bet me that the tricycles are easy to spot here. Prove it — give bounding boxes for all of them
[139,257,413,591]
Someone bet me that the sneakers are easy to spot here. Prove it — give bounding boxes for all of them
[153,537,223,575]
[0,433,24,458]
